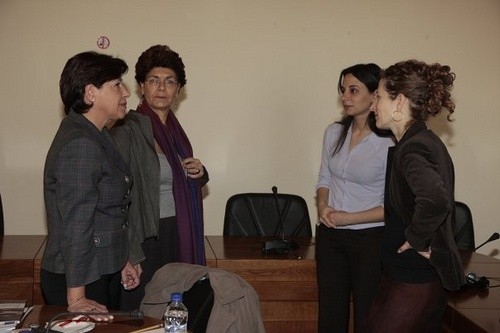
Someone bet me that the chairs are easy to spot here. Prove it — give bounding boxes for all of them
[139,262,272,333]
[222,192,312,260]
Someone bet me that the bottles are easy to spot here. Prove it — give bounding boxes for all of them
[164,293,188,333]
[463,271,480,283]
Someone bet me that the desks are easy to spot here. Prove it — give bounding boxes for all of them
[0,235,500,333]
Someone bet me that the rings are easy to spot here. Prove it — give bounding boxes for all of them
[197,169,200,173]
[90,308,95,312]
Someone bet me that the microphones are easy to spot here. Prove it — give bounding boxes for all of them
[45,310,144,333]
[264,186,290,256]
[472,233,499,252]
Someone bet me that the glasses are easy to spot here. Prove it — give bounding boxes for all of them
[145,75,180,90]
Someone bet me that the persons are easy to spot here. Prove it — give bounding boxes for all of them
[369,60,464,333]
[110,44,209,310]
[315,62,398,333]
[40,50,140,322]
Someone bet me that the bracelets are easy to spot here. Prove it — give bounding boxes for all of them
[69,296,86,305]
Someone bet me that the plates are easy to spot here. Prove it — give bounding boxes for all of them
[45,320,95,333]
